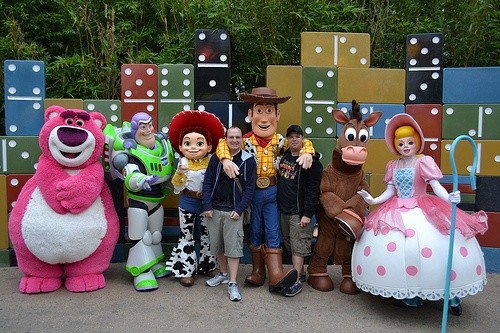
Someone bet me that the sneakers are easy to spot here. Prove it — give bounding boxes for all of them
[299,271,307,282]
[282,280,302,297]
[228,282,242,301]
[206,272,229,287]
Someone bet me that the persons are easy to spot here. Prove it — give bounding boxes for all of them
[102,112,175,290]
[216,87,315,292]
[201,126,257,301]
[277,124,323,297]
[165,110,225,286]
[350,112,489,315]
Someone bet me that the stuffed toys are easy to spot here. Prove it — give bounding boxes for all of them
[8,105,120,294]
[307,99,383,294]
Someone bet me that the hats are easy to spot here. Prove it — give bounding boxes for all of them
[286,125,303,137]
